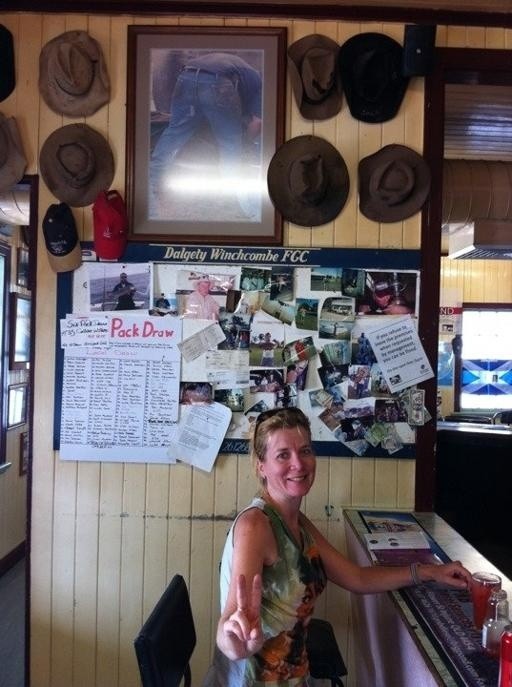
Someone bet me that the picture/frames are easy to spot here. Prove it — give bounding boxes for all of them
[121,20,289,247]
[0,238,31,479]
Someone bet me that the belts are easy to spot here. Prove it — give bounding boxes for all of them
[183,68,215,75]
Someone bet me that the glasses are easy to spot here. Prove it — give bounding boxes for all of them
[253,408,303,451]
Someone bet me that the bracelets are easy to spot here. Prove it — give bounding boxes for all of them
[409,560,424,586]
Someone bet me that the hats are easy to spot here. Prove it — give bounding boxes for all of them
[373,281,394,299]
[1,111,28,195]
[334,32,409,124]
[36,30,113,119]
[1,23,17,102]
[266,134,351,227]
[285,34,343,122]
[358,144,433,224]
[39,123,129,274]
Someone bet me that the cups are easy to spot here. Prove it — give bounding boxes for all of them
[469,569,501,628]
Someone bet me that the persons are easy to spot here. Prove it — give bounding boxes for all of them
[359,279,414,314]
[185,275,228,319]
[287,359,309,388]
[356,332,367,355]
[149,51,261,220]
[202,406,474,687]
[155,293,170,308]
[111,272,137,309]
[258,332,280,366]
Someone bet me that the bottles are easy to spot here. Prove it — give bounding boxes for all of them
[480,585,511,656]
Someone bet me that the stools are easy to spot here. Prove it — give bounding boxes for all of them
[306,618,349,687]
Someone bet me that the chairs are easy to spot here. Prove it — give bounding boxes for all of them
[440,408,512,427]
[127,572,203,686]
